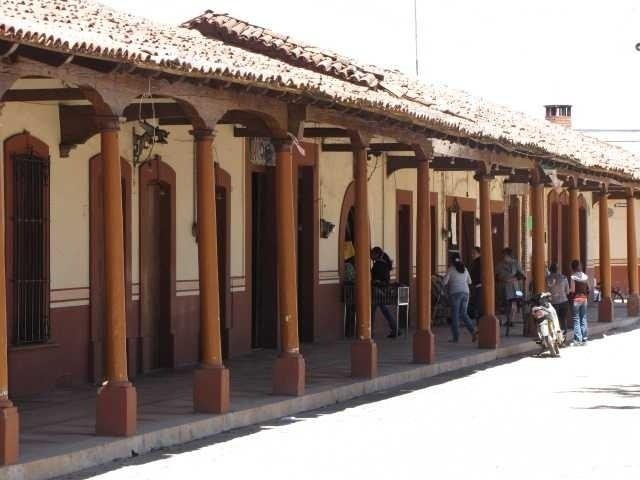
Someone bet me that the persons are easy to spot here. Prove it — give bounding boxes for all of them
[493,247,527,328]
[563,259,591,344]
[442,251,480,344]
[467,246,482,334]
[362,245,404,339]
[545,263,570,336]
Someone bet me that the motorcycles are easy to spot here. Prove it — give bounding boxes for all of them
[516,289,568,356]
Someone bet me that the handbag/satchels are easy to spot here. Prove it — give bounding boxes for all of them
[343,241,356,261]
[516,270,526,280]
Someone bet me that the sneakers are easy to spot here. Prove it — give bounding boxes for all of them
[387,327,402,338]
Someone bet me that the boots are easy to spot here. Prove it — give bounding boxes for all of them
[471,328,479,343]
[448,335,458,344]
